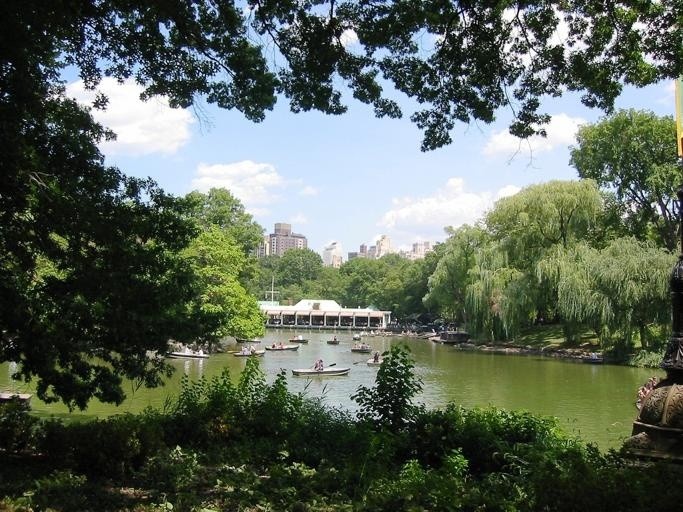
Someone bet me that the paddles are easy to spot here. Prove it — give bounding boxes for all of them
[323,364,336,369]
[353,358,373,365]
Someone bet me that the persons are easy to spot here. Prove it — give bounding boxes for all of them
[316,360,323,371]
[250,344,256,354]
[269,317,367,327]
[278,342,284,349]
[334,336,337,340]
[271,343,276,348]
[590,350,598,359]
[373,352,380,363]
[242,346,250,355]
[314,360,320,369]
[636,376,659,407]
[355,342,371,349]
[196,347,203,355]
[184,345,191,353]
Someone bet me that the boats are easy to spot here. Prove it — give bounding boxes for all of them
[326,341,340,344]
[353,331,397,341]
[292,367,351,376]
[289,339,308,344]
[233,350,266,357]
[167,349,210,361]
[234,338,261,344]
[265,345,300,351]
[365,358,384,367]
[0,392,32,411]
[583,357,604,364]
[351,348,373,353]
